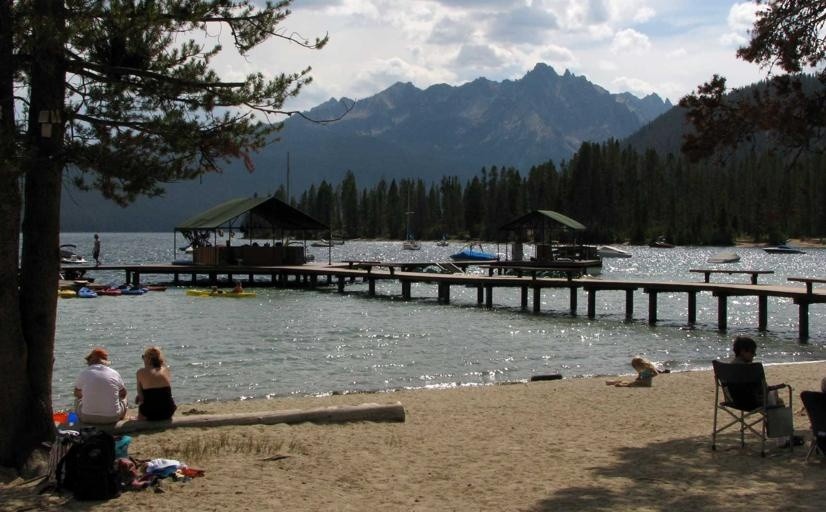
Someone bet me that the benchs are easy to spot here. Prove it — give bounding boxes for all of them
[341,260,404,275]
[479,265,579,281]
[689,269,775,285]
[787,276,826,294]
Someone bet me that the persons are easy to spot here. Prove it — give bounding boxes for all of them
[605,354,659,388]
[91,233,101,266]
[73,347,129,425]
[210,285,218,292]
[729,335,796,449]
[134,346,177,422]
[227,280,244,293]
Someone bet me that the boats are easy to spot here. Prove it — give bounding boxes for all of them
[320,238,345,245]
[59,242,89,279]
[595,245,633,259]
[449,242,497,262]
[649,239,674,248]
[57,283,168,299]
[437,236,449,247]
[311,242,334,248]
[762,243,807,255]
[553,244,603,276]
[708,252,740,263]
[185,286,256,299]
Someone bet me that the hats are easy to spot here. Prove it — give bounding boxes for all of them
[84,348,108,360]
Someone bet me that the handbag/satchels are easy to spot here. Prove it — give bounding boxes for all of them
[55,427,136,502]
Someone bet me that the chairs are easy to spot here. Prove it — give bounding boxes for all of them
[711,358,826,462]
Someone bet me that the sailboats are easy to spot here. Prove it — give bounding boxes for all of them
[403,188,421,250]
